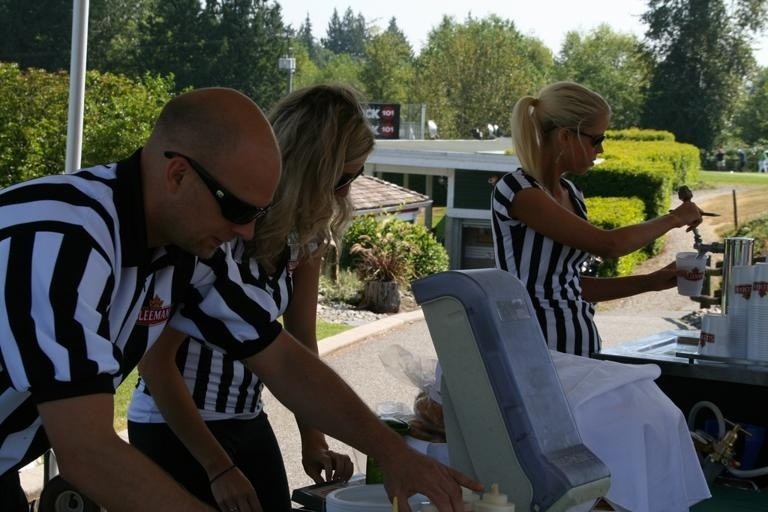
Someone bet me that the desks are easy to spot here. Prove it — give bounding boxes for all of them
[292,474,363,511]
[596,326,768,469]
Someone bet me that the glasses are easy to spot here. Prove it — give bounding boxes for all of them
[336,167,366,192]
[162,149,273,225]
[572,127,605,148]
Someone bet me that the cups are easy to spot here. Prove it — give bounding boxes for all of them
[674,251,708,297]
[697,262,768,366]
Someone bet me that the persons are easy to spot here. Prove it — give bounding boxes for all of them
[0,84,486,511]
[715,149,727,172]
[735,149,747,172]
[489,80,705,360]
[125,84,379,511]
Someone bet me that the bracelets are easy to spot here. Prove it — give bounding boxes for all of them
[208,462,236,488]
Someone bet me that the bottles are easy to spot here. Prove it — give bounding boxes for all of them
[414,482,518,512]
[365,414,408,483]
